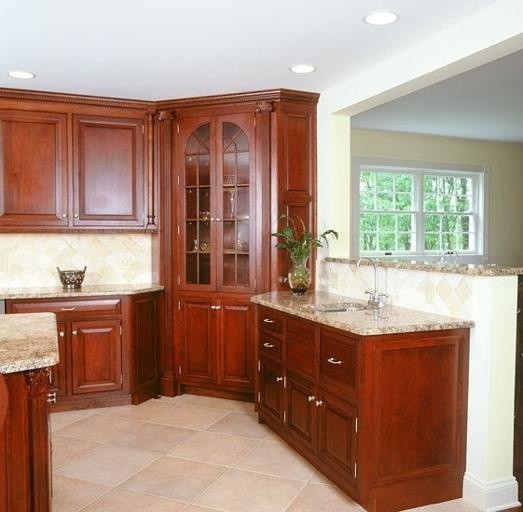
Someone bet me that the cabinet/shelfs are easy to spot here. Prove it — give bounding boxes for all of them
[156,88,321,402]
[285,313,470,512]
[0,87,156,234]
[5,291,157,413]
[0,368,56,512]
[257,303,285,441]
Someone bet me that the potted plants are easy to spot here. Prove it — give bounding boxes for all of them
[271,214,339,296]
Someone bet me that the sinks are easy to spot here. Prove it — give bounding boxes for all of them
[304,302,373,313]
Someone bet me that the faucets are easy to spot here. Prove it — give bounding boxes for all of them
[356,257,377,301]
